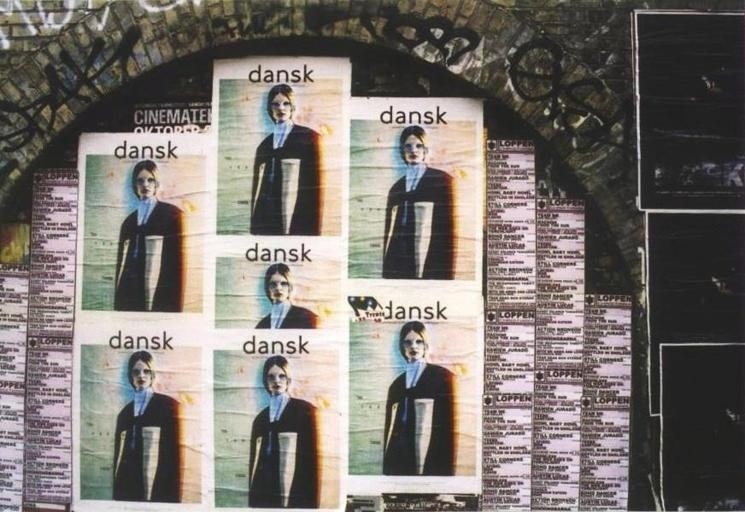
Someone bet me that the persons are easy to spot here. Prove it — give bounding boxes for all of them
[381,126,459,280]
[112,350,184,503]
[249,84,325,236]
[253,263,321,329]
[249,356,323,508]
[114,160,186,310]
[383,320,460,475]
